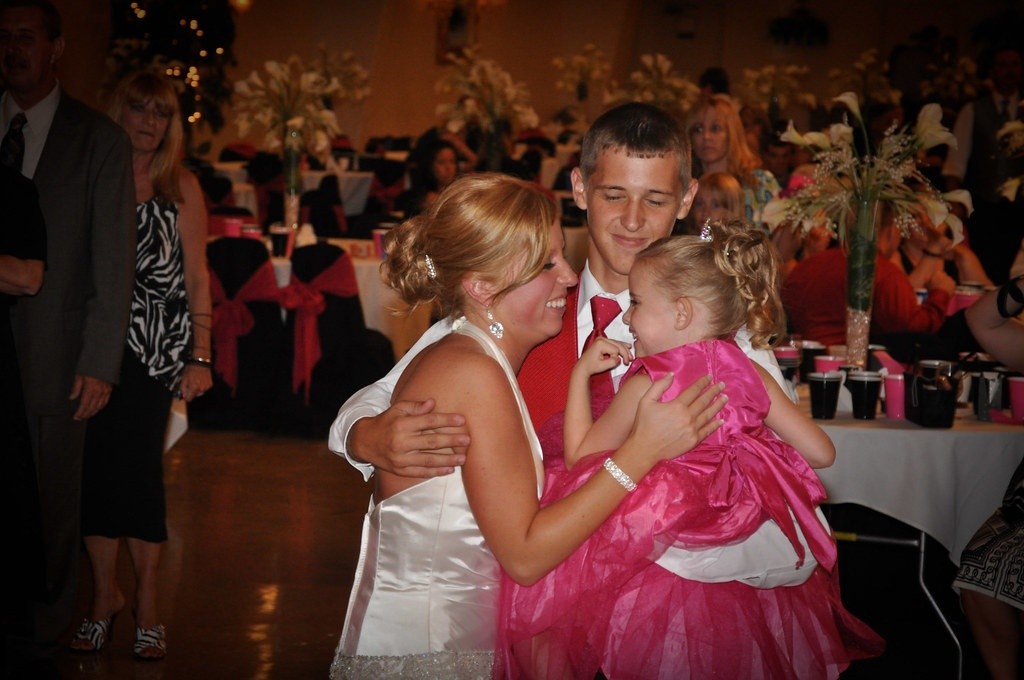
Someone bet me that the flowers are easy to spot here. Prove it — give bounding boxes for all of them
[233,46,974,307]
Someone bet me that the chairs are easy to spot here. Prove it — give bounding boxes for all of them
[194,124,1024,439]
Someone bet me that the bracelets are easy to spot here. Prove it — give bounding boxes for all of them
[600,457,638,494]
[923,247,942,258]
[188,313,213,368]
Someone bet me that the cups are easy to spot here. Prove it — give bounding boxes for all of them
[838,365,859,391]
[373,230,392,260]
[916,289,927,305]
[848,371,882,421]
[803,341,829,383]
[1009,377,1024,419]
[972,372,1010,421]
[946,284,998,316]
[815,355,847,373]
[772,347,800,381]
[268,226,295,260]
[223,218,261,238]
[809,372,842,420]
[884,374,905,420]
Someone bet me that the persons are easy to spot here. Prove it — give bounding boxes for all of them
[204,148,345,290]
[783,53,1024,372]
[0,0,214,680]
[699,67,732,101]
[327,99,884,679]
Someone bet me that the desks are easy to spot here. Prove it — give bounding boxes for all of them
[214,161,373,236]
[263,239,432,364]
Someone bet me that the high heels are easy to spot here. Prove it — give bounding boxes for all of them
[131,606,167,660]
[70,612,117,655]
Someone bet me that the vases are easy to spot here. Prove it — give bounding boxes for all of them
[848,198,878,369]
[285,128,302,229]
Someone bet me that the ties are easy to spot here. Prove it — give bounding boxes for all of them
[577,295,622,419]
[0,112,28,172]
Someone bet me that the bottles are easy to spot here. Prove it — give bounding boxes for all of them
[905,360,959,429]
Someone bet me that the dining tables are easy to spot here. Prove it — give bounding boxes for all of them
[789,382,1024,680]
